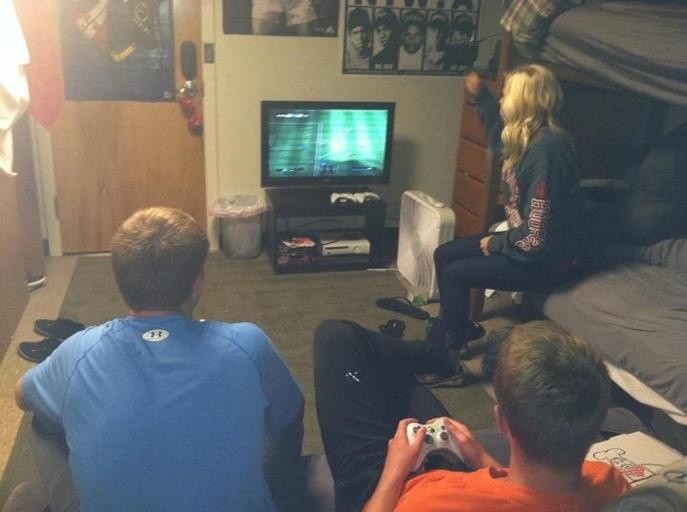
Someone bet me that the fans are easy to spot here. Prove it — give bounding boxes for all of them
[394,190,458,305]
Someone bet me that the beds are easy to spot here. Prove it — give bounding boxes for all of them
[509,0,684,466]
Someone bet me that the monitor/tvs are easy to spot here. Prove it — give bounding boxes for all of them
[261,100,395,188]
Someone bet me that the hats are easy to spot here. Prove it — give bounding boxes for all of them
[348,9,477,32]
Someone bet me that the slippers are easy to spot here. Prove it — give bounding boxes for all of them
[378,319,406,337]
[376,296,430,320]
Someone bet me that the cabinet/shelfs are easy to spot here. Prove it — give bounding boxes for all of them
[452,76,515,322]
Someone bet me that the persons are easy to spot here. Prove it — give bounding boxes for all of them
[345,7,477,72]
[312,317,633,512]
[250,0,317,35]
[399,64,584,387]
[14,206,306,511]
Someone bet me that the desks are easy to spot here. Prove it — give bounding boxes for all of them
[263,188,388,274]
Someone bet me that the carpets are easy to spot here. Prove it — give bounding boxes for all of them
[1,247,661,510]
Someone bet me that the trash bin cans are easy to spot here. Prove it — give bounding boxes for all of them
[212,194,268,260]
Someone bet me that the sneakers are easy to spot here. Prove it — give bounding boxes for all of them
[418,319,472,389]
[18,339,63,362]
[33,318,85,340]
